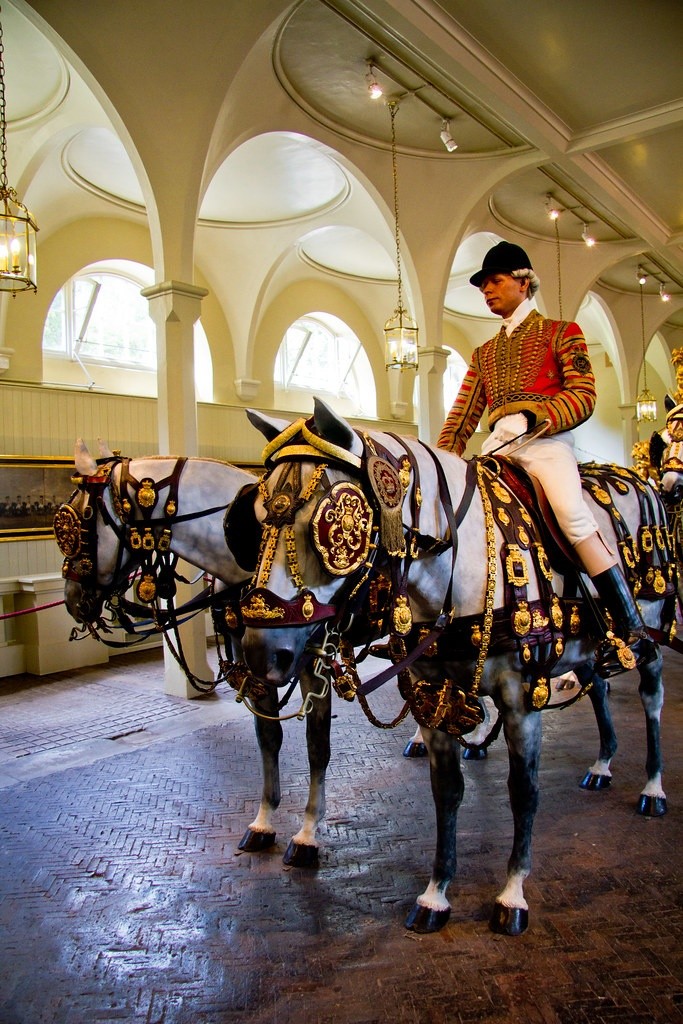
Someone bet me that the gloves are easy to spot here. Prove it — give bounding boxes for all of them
[494,414,528,443]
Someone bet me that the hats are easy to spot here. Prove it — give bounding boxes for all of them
[469,241,534,286]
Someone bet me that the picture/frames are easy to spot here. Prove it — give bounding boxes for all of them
[0,451,82,542]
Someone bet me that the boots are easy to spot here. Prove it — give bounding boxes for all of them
[593,564,659,676]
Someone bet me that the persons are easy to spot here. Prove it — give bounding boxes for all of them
[435,242,652,664]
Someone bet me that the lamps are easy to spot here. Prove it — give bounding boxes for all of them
[437,118,459,154]
[635,261,658,424]
[2,9,40,299]
[364,68,384,101]
[659,282,671,303]
[579,222,595,246]
[636,267,646,286]
[382,91,421,374]
[543,195,560,222]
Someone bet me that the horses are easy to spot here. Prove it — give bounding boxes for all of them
[238,396,668,935]
[64,436,489,869]
